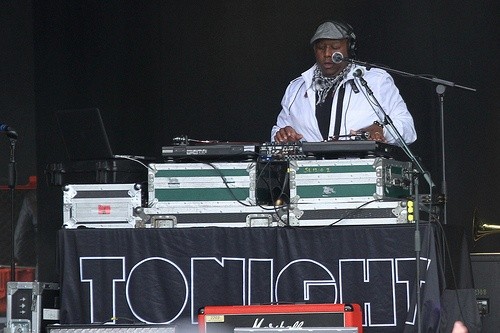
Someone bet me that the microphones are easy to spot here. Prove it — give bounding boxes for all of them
[0,122,19,138]
[331,51,343,64]
[353,67,366,78]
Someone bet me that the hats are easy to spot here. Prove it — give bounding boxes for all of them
[310,20,355,42]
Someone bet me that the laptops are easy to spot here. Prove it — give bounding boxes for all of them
[57,108,156,160]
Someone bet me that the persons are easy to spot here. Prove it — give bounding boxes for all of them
[271,19,417,147]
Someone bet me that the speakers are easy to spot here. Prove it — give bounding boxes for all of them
[469,251,500,333]
